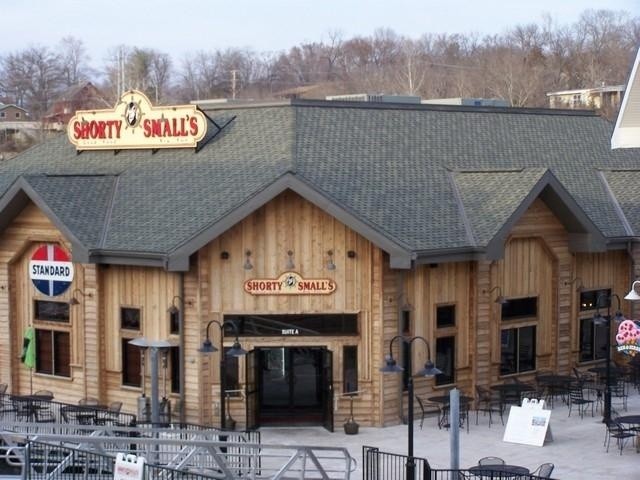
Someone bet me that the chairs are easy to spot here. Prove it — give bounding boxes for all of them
[594,408,639,455]
[460,454,555,480]
[0,383,123,426]
[413,357,640,432]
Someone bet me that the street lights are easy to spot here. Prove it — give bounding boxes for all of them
[593,293,626,423]
[623,280,640,300]
[195,319,248,473]
[380,336,445,480]
